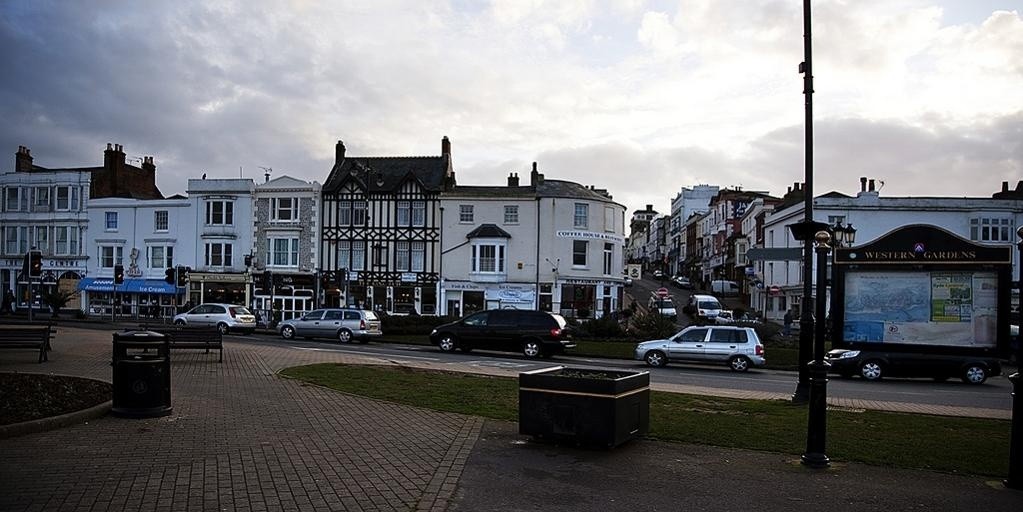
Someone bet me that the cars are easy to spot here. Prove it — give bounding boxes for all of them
[823,339,1005,386]
[623,263,764,327]
[171,302,258,337]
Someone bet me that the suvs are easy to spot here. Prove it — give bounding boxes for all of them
[272,305,384,346]
[427,307,578,360]
[632,322,767,373]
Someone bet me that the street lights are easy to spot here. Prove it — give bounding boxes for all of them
[720,245,728,301]
[832,219,857,248]
[657,240,668,328]
[350,159,386,306]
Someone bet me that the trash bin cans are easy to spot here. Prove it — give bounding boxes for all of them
[110,330,173,419]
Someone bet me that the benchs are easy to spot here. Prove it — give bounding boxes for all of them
[139,324,222,363]
[0,327,50,363]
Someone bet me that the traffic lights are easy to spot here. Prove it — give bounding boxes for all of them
[322,274,330,292]
[333,268,346,292]
[175,265,190,288]
[114,264,124,284]
[261,271,274,290]
[28,250,43,280]
[165,269,173,286]
